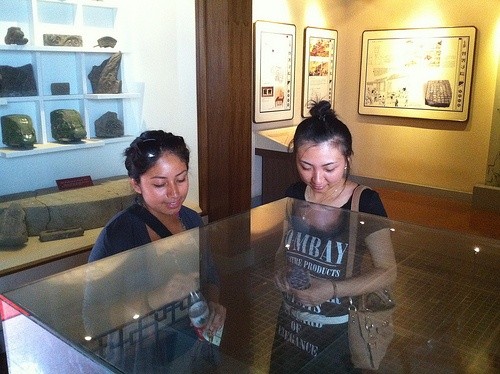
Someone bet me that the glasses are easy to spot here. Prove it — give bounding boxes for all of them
[140,135,186,159]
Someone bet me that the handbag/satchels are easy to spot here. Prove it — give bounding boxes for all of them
[348,289,395,372]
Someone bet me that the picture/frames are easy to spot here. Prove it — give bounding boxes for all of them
[358,26,477,123]
[252,19,297,123]
[302,26,338,119]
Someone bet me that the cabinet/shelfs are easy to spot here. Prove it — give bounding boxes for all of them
[0,0,138,196]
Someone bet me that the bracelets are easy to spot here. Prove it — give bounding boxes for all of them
[328,279,337,299]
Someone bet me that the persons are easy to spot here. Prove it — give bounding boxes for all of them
[84,130,227,374]
[269,101,396,374]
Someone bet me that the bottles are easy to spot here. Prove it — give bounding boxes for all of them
[188,288,211,341]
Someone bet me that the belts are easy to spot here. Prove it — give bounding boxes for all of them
[282,299,349,325]
